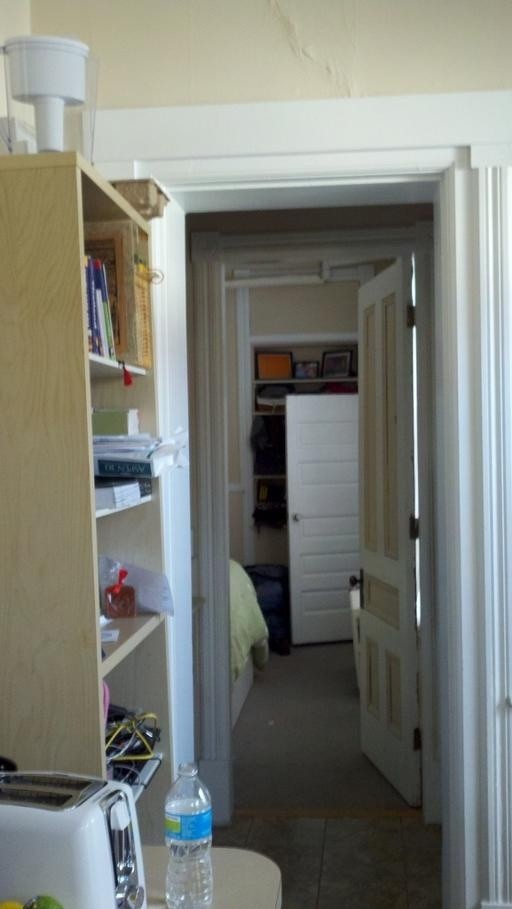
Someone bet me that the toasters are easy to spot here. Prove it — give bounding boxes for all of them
[0,770,148,909]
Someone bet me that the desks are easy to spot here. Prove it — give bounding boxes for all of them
[142,845,282,909]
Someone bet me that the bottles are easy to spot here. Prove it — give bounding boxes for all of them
[161,760,215,909]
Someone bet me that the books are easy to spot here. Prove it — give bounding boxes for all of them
[84,254,117,360]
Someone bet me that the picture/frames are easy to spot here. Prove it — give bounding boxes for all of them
[256,349,353,379]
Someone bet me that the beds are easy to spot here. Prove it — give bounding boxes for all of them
[230,559,270,730]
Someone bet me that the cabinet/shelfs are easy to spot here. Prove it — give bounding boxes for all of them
[0,152,174,844]
[247,331,358,568]
